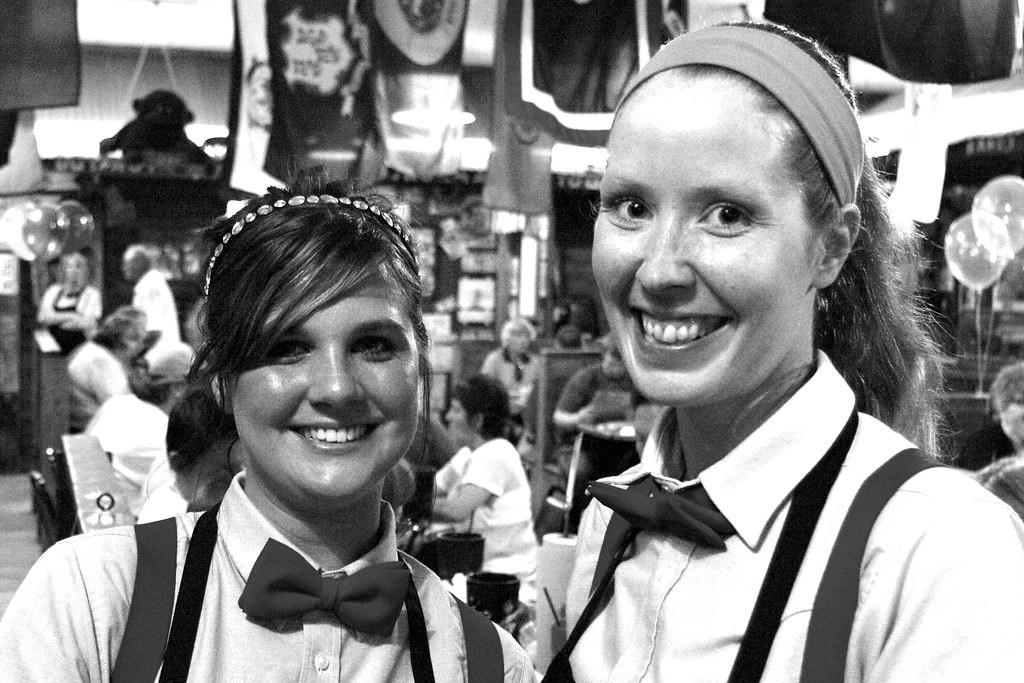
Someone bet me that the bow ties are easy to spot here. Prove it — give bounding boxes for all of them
[585,479,736,551]
[239,538,411,637]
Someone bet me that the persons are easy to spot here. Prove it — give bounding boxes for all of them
[553,331,642,530]
[520,321,579,438]
[434,368,539,634]
[35,244,243,526]
[562,15,1024,682]
[978,357,1024,523]
[2,162,540,683]
[474,317,541,444]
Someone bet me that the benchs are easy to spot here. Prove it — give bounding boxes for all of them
[520,346,604,540]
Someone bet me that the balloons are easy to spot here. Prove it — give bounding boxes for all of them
[23,205,70,263]
[968,174,1024,263]
[2,200,43,264]
[55,199,95,256]
[942,204,1006,296]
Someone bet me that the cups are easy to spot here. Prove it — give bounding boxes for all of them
[538,533,579,675]
[467,574,519,644]
[550,621,566,661]
[442,533,484,582]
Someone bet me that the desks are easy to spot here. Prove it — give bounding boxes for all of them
[563,423,646,539]
